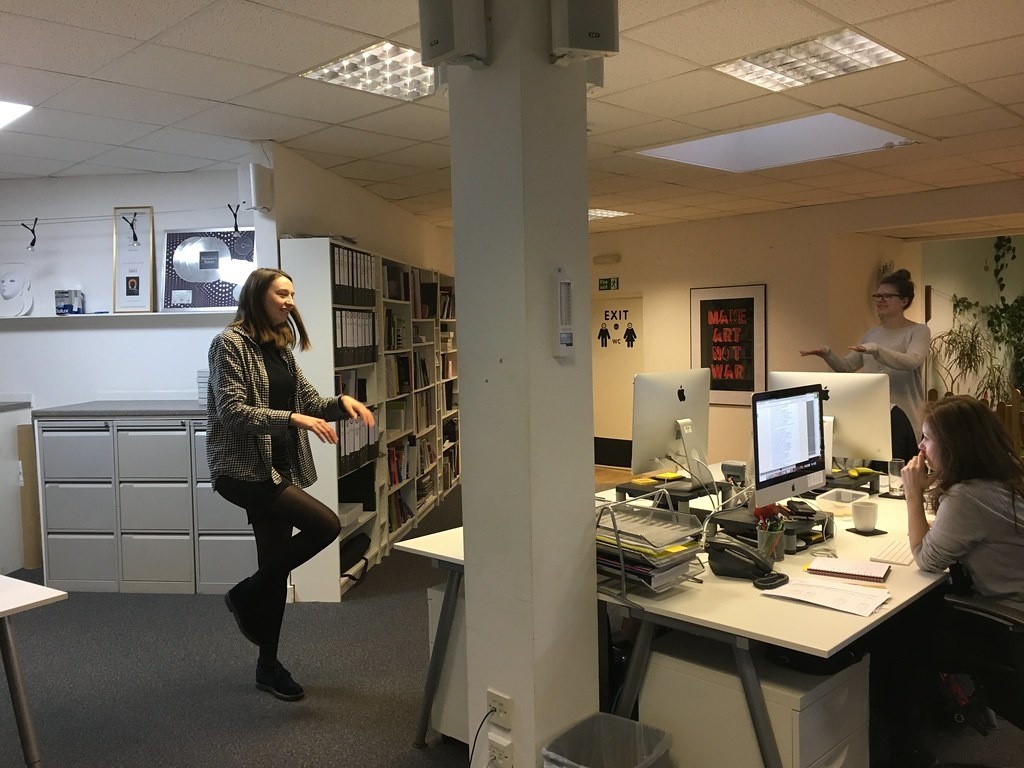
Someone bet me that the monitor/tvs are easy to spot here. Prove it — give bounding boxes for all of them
[750,383,827,521]
[630,367,713,491]
[767,372,891,478]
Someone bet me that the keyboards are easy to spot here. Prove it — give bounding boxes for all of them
[869,535,916,565]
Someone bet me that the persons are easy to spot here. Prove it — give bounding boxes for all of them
[883,394,1024,768]
[209,268,374,700]
[796,266,930,471]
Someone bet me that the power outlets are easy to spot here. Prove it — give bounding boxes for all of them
[19,460,24,487]
[485,686,515,732]
[487,733,513,768]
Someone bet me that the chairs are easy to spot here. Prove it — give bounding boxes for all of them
[943,593,1024,730]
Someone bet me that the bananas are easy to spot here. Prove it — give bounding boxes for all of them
[848,467,877,478]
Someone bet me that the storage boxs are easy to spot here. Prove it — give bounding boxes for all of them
[815,487,869,518]
[339,503,363,526]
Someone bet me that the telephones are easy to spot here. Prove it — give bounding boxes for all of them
[707,533,774,577]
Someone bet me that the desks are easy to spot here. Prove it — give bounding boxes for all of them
[393,466,950,768]
[0,572,68,768]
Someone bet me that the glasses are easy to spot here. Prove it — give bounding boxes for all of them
[872,294,903,303]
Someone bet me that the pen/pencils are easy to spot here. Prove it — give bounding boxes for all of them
[777,502,796,514]
[757,513,784,531]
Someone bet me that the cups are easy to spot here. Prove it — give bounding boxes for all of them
[852,502,878,532]
[888,459,905,497]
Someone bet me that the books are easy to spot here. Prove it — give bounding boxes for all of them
[806,556,892,583]
[596,511,701,589]
[380,257,457,534]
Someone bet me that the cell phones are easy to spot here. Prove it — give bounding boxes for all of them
[787,500,816,515]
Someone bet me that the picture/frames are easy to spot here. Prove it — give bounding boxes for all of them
[690,285,768,407]
[160,227,258,312]
[114,205,153,314]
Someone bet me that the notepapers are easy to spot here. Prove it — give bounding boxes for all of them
[631,479,660,485]
[656,473,682,480]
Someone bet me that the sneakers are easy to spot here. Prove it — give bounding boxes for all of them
[256,660,305,700]
[225,579,266,648]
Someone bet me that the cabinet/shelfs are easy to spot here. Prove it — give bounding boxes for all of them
[31,400,259,596]
[425,579,469,743]
[639,636,870,768]
[279,239,461,603]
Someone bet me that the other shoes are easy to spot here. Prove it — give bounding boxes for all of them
[889,748,940,768]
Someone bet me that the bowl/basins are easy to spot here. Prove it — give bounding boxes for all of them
[816,488,870,517]
